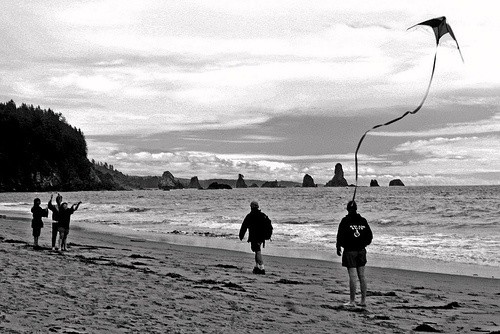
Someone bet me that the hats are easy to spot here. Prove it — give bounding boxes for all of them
[347,201,357,209]
[250,202,259,208]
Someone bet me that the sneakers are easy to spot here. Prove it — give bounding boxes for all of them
[253,267,259,273]
[255,268,265,274]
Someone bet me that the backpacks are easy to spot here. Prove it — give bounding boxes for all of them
[260,212,273,240]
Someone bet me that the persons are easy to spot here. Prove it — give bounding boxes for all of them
[335,201,373,307]
[47,194,64,251]
[238,201,273,275]
[56,193,82,251]
[31,198,49,250]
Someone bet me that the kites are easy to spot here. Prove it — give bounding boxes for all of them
[349,16,464,203]
